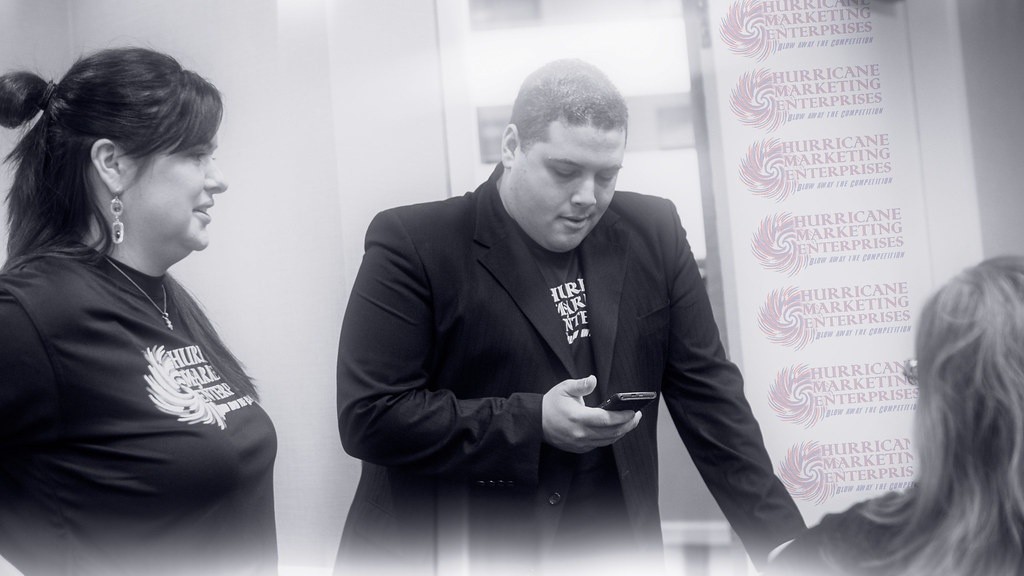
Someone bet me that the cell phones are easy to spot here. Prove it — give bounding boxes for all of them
[596,391,658,413]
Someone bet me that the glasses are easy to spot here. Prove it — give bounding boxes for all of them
[902,358,923,386]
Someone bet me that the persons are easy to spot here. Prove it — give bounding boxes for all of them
[0,45,277,576]
[763,254,1024,575]
[337,59,806,576]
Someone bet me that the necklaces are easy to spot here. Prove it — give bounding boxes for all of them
[103,256,173,330]
